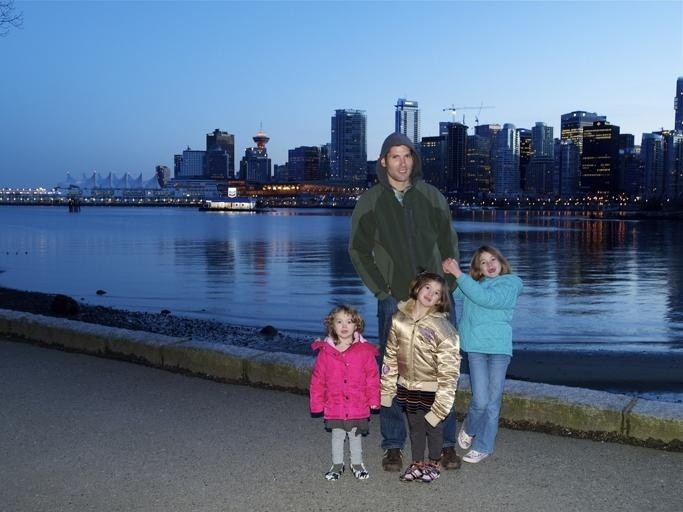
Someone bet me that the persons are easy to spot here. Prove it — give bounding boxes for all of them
[348,132,461,472]
[442,246,523,463]
[380,266,461,483]
[310,304,381,482]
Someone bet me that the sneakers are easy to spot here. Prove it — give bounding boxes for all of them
[350,462,369,479]
[442,450,461,469]
[463,450,489,463]
[325,463,345,482]
[399,462,425,482]
[420,462,440,482]
[381,449,403,472]
[457,419,473,450]
